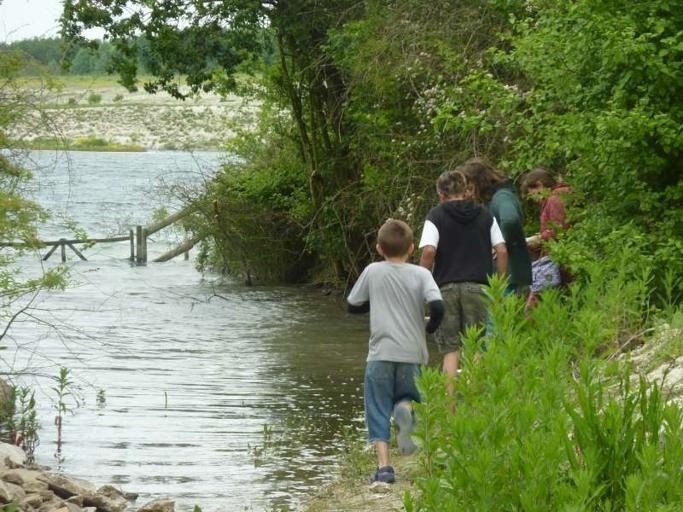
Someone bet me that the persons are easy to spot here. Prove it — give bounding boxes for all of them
[416,159,580,419]
[347,221,445,481]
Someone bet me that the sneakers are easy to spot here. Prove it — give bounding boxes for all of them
[368,465,395,493]
[392,404,416,458]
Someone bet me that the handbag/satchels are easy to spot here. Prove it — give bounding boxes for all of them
[527,256,561,293]
